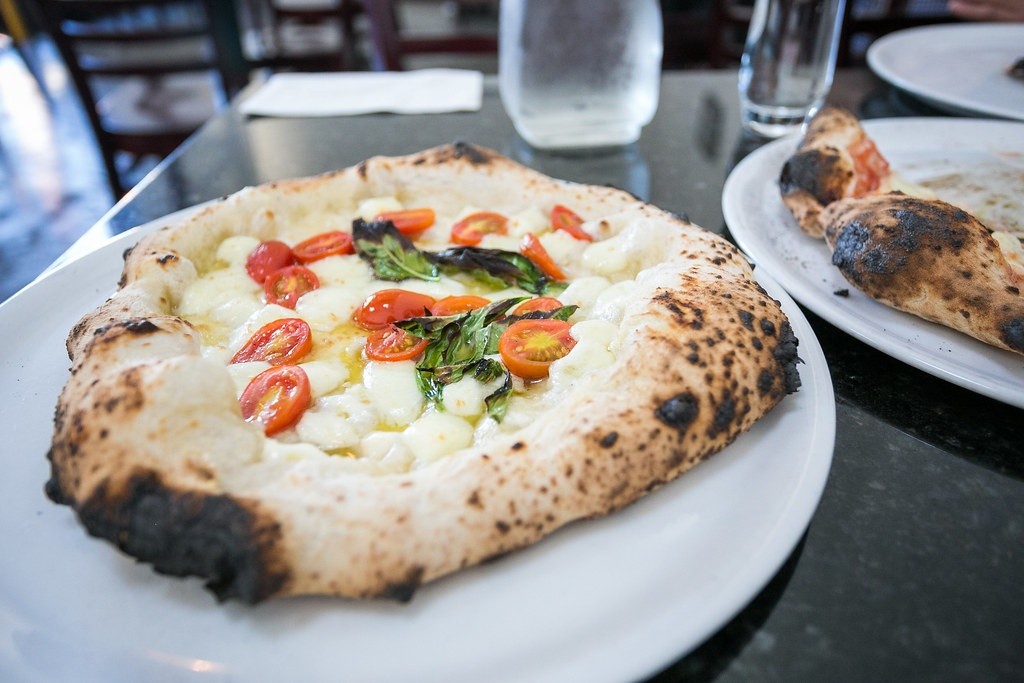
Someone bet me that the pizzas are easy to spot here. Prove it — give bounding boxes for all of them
[45,138,801,605]
[777,103,1024,358]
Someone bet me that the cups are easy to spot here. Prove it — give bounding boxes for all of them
[738,0,847,139]
[498,0,664,149]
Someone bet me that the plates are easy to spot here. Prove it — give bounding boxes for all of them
[866,23,1024,121]
[722,118,1024,408]
[1,195,837,683]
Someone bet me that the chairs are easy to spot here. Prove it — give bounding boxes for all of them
[19,0,500,204]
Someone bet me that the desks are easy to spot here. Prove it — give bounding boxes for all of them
[31,71,1024,683]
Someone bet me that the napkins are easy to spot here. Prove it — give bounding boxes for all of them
[236,67,485,117]
